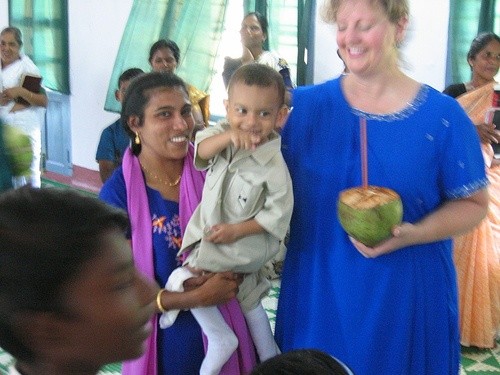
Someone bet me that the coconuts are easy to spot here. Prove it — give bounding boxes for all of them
[337,185,403,246]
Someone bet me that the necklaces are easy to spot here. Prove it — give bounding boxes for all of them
[140,163,182,186]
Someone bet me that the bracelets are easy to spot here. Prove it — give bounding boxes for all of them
[157,288,166,312]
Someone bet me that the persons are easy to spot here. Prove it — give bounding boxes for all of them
[275,0,489,375]
[148,40,210,142]
[96,67,147,183]
[222,12,293,91]
[97,71,260,375]
[250,349,353,375]
[0,184,160,375]
[177,63,294,375]
[441,32,500,348]
[0,26,48,192]
